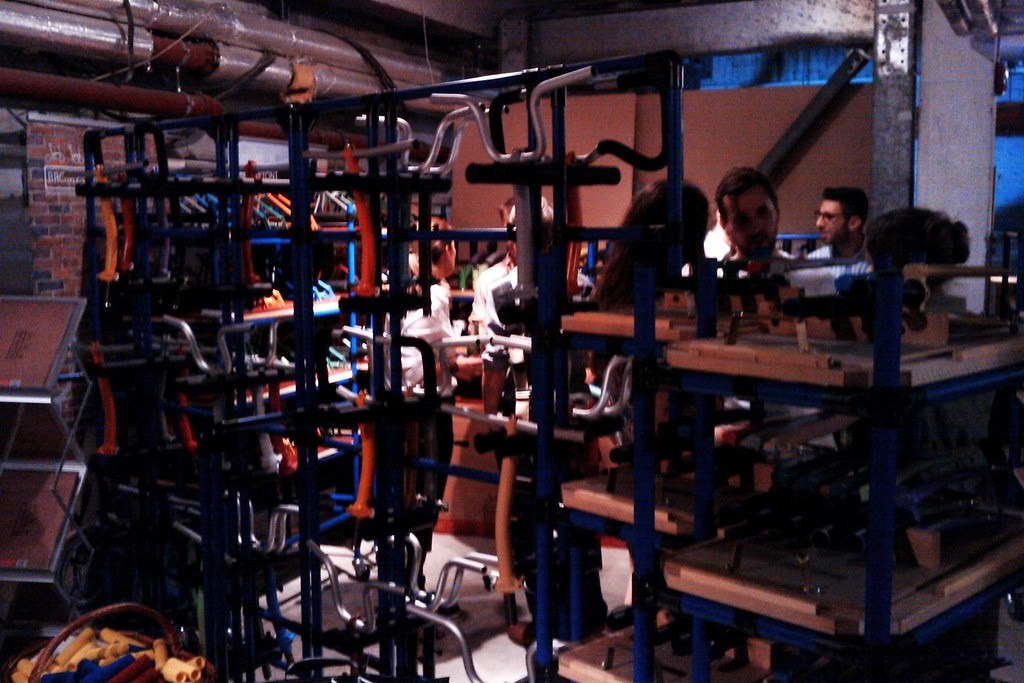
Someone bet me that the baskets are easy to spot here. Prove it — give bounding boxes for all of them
[0,602,217,683]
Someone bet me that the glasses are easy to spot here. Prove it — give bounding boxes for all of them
[815,210,842,224]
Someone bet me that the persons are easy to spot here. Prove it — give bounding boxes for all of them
[798,186,877,297]
[378,166,808,646]
[860,203,971,309]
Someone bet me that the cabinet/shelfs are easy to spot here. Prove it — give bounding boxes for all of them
[58,50,1024,683]
